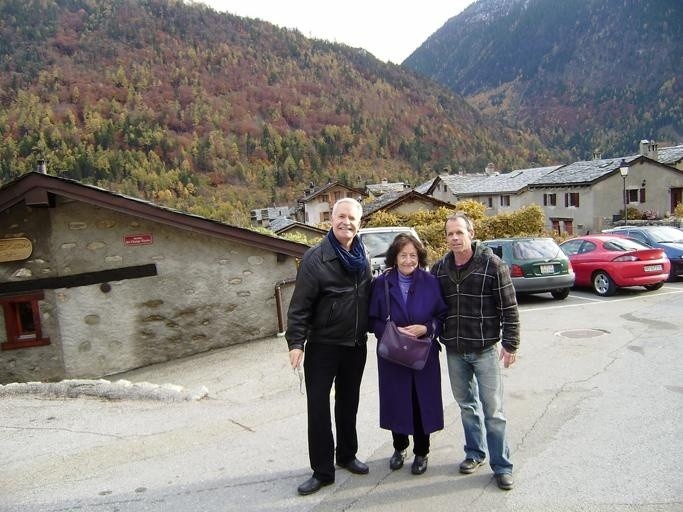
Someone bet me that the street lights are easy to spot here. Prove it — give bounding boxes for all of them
[618,159,630,226]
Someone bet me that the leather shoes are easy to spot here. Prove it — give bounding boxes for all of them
[298,474,334,496]
[336,457,369,475]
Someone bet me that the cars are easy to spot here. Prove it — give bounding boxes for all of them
[357,226,430,277]
[483,226,683,300]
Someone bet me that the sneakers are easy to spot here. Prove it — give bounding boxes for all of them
[390,449,407,471]
[460,457,486,475]
[496,472,514,490]
[411,456,428,475]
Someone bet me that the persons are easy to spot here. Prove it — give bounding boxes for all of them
[662,211,674,226]
[640,210,656,226]
[429,214,520,492]
[368,232,448,476]
[283,196,372,496]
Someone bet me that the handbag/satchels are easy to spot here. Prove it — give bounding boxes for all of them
[376,272,438,370]
